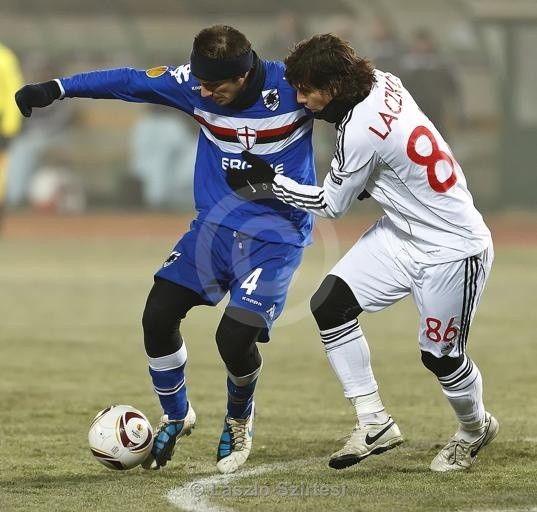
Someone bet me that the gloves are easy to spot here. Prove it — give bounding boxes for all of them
[226,151,277,203]
[15,81,61,118]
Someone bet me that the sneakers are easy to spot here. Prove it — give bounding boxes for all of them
[328,414,404,470]
[430,411,500,471]
[216,399,254,474]
[140,400,197,470]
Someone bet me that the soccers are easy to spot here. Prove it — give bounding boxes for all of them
[88,404,155,469]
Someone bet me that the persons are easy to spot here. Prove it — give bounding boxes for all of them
[14,21,319,478]
[1,42,26,208]
[223,31,500,475]
